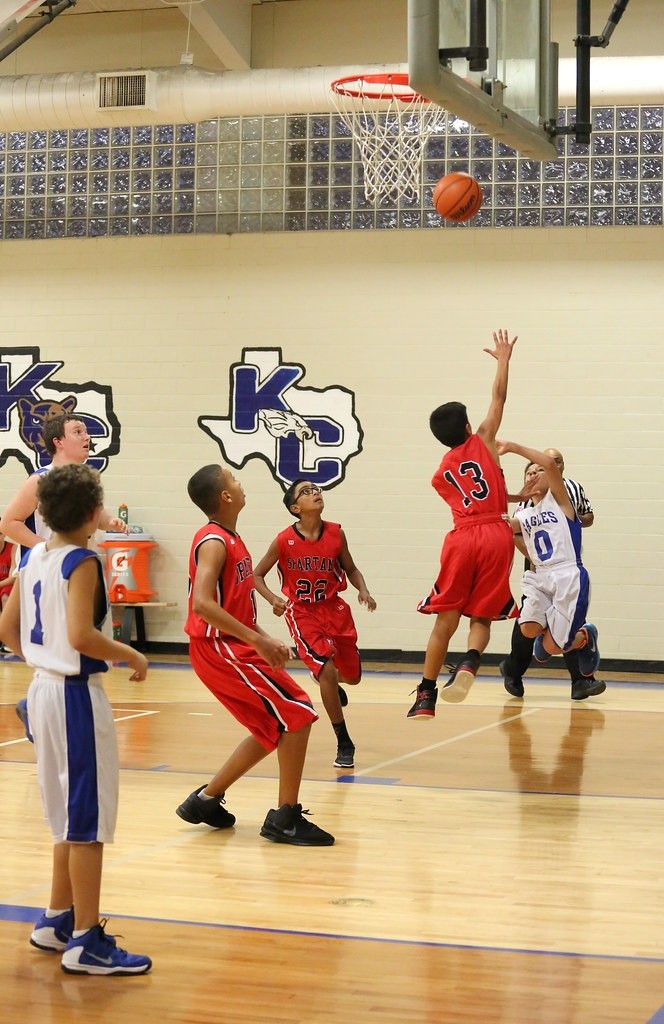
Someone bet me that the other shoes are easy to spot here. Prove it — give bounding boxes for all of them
[0,645,26,663]
[16,697,32,742]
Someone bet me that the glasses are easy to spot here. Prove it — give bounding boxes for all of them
[293,487,323,504]
[64,425,87,438]
[93,500,105,510]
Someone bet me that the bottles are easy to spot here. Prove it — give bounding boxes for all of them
[112,619,122,640]
[118,504,128,525]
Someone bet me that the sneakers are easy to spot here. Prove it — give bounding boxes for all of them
[63,916,150,974]
[175,783,236,829]
[441,657,477,704]
[259,804,334,846]
[499,660,525,699]
[534,632,552,664]
[571,675,606,699]
[310,671,348,708]
[580,622,600,679]
[406,684,437,718]
[28,902,75,951]
[334,741,356,768]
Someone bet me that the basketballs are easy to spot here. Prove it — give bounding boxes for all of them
[433,171,483,222]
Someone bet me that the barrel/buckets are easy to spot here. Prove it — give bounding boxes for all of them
[96,533,158,602]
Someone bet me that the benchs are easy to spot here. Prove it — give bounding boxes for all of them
[111,602,177,652]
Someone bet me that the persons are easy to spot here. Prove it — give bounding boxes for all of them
[253,479,376,768]
[0,413,129,743]
[0,517,18,652]
[492,439,600,677]
[174,464,335,846]
[408,328,518,721]
[498,448,607,700]
[0,464,153,976]
